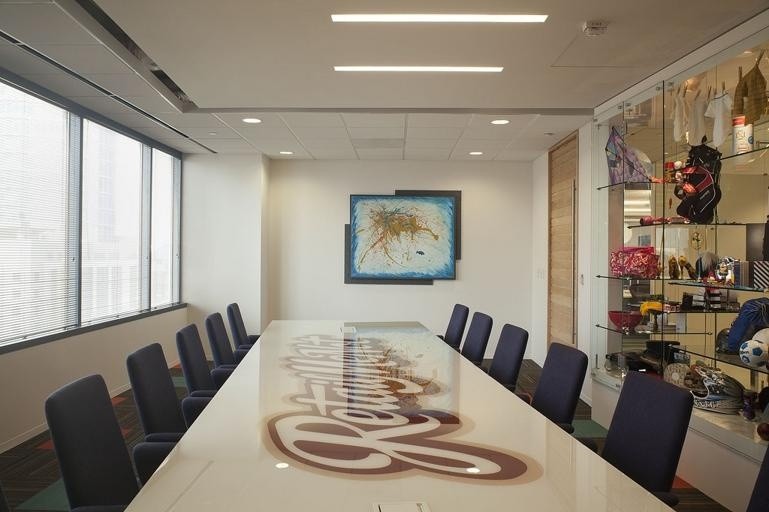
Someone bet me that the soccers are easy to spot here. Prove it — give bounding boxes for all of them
[739,341,769,368]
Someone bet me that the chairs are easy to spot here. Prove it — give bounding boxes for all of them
[43,300,261,510]
[743,447,769,512]
[434,303,695,507]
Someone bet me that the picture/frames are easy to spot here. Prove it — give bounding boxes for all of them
[342,321,460,425]
[348,193,457,282]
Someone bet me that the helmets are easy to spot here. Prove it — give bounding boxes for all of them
[690,365,746,416]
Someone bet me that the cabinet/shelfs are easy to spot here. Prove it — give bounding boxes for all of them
[591,24,769,466]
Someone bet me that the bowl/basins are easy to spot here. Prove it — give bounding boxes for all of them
[607,309,644,330]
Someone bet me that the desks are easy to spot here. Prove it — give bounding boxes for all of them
[124,319,673,512]
